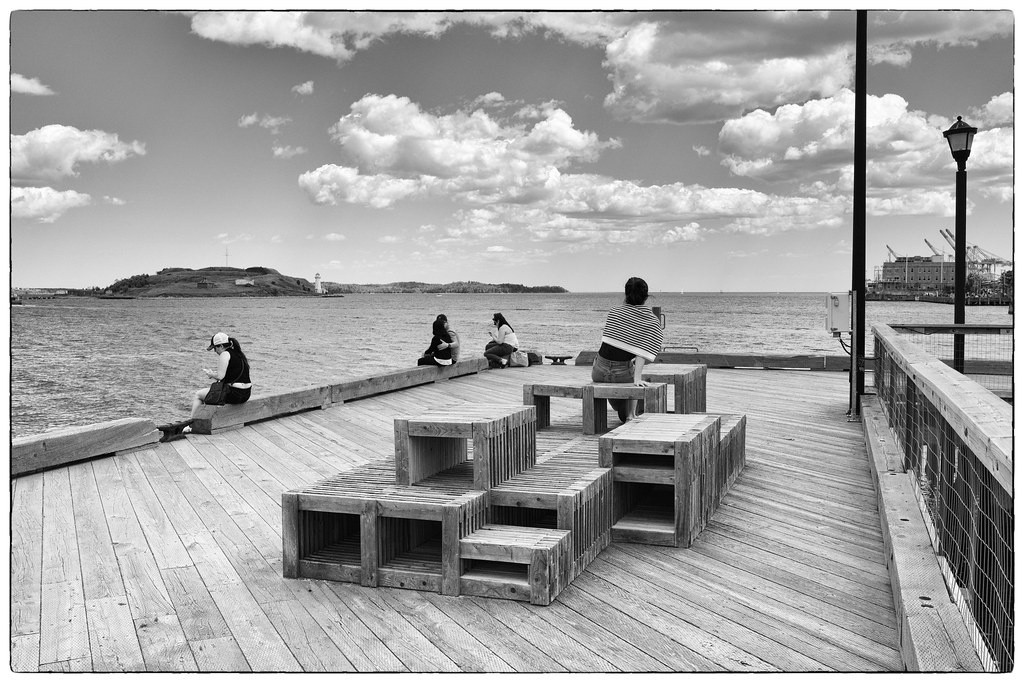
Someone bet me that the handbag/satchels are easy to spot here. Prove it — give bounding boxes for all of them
[204,379,230,406]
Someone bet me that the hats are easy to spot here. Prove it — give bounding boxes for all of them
[438,314,448,322]
[208,332,230,352]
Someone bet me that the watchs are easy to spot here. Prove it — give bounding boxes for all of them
[448,344,451,348]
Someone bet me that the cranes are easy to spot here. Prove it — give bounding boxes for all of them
[939,227,1013,300]
[886,244,902,259]
[924,238,955,263]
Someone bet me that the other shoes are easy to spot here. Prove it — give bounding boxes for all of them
[501,359,508,369]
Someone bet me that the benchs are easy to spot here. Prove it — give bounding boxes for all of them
[394,406,537,491]
[598,412,722,468]
[641,362,707,414]
[523,381,667,436]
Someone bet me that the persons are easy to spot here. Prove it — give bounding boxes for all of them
[184,332,252,432]
[418,314,460,366]
[484,312,519,369]
[591,277,663,424]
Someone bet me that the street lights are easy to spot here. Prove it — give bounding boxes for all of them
[942,117,978,374]
